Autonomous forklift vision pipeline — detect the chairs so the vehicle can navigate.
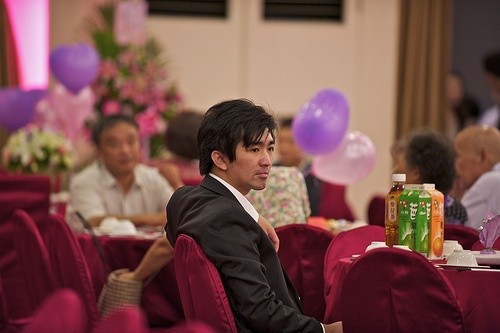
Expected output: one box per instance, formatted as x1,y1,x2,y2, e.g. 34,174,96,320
0,164,500,333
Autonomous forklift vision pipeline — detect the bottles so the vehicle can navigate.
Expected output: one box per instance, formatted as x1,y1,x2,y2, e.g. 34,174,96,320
399,183,444,260
385,174,406,247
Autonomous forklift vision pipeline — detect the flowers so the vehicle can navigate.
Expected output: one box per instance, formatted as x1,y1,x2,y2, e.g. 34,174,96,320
2,4,189,191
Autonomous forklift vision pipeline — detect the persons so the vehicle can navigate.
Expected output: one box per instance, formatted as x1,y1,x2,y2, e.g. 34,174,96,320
64,114,175,234
391,51,500,232
273,117,320,217
164,100,343,333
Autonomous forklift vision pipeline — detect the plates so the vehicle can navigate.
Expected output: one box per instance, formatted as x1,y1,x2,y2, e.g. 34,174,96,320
438,264,490,269
468,251,500,264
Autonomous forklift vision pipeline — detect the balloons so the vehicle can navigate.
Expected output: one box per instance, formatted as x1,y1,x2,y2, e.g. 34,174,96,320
310,131,376,185
0,43,100,134
292,89,349,156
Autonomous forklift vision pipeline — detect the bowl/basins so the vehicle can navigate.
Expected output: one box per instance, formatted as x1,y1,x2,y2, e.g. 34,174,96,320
446,249,477,266
365,242,388,253
99,217,137,234
443,240,463,256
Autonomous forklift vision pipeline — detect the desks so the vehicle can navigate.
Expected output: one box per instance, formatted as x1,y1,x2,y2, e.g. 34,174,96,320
82,227,182,326
323,257,500,333
